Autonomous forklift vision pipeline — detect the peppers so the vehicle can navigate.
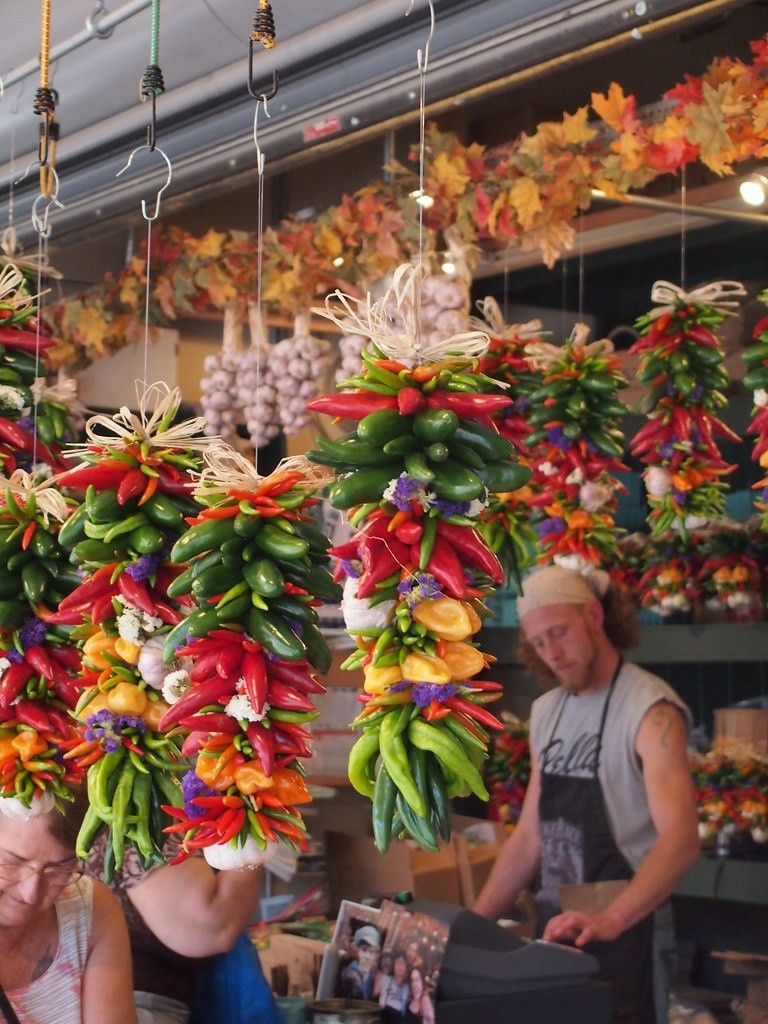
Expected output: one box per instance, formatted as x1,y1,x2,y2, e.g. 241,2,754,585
0,293,768,885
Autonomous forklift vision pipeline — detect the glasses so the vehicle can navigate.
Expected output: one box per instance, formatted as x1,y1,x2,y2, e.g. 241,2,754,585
0,858,85,887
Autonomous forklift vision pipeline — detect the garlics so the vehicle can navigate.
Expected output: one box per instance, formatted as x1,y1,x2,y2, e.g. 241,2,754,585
578,481,608,513
199,278,462,447
644,466,675,496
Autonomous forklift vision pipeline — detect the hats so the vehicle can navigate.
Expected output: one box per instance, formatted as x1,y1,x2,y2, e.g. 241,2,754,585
355,925,379,949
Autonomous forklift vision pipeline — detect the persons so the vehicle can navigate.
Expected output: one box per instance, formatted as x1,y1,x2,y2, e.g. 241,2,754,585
0,782,139,1024
470,554,702,1024
340,925,435,1024
78,822,263,1024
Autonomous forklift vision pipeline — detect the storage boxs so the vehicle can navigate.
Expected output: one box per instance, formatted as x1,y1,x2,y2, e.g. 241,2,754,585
328,808,510,924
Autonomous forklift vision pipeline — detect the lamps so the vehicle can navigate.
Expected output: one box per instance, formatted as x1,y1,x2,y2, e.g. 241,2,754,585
738,165,768,205
